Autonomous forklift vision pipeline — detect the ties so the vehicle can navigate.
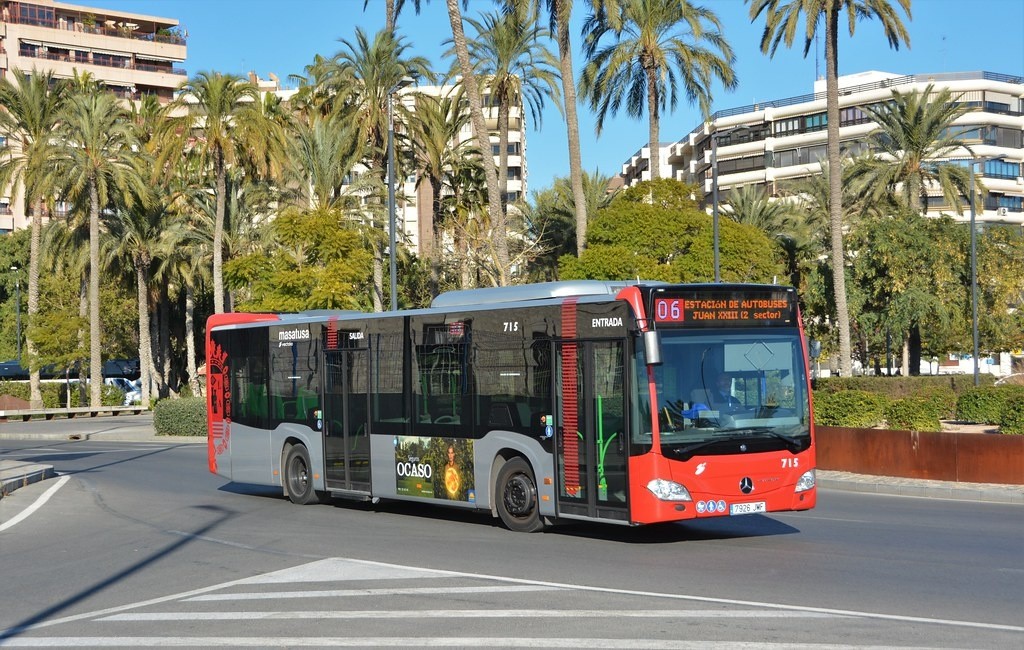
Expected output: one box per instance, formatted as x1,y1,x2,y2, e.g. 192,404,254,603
725,395,730,403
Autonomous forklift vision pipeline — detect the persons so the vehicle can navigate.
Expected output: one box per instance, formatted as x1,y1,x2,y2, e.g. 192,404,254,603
707,370,741,413
444,445,460,497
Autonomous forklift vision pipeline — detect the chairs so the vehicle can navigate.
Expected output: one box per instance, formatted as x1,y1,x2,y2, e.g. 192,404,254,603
405,416,458,424
488,403,513,427
249,382,319,420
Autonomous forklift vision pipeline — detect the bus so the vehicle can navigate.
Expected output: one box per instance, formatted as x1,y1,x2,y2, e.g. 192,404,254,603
207,280,821,535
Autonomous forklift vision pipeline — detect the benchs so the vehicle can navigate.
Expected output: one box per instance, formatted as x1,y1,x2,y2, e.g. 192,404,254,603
0,405,149,422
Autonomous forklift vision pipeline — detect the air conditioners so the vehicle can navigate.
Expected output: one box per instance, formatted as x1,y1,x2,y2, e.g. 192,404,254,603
998,208,1008,215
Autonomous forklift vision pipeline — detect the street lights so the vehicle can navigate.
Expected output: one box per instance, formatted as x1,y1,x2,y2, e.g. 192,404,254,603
970,153,1010,384
710,124,750,283
11,266,21,360
385,76,416,311
